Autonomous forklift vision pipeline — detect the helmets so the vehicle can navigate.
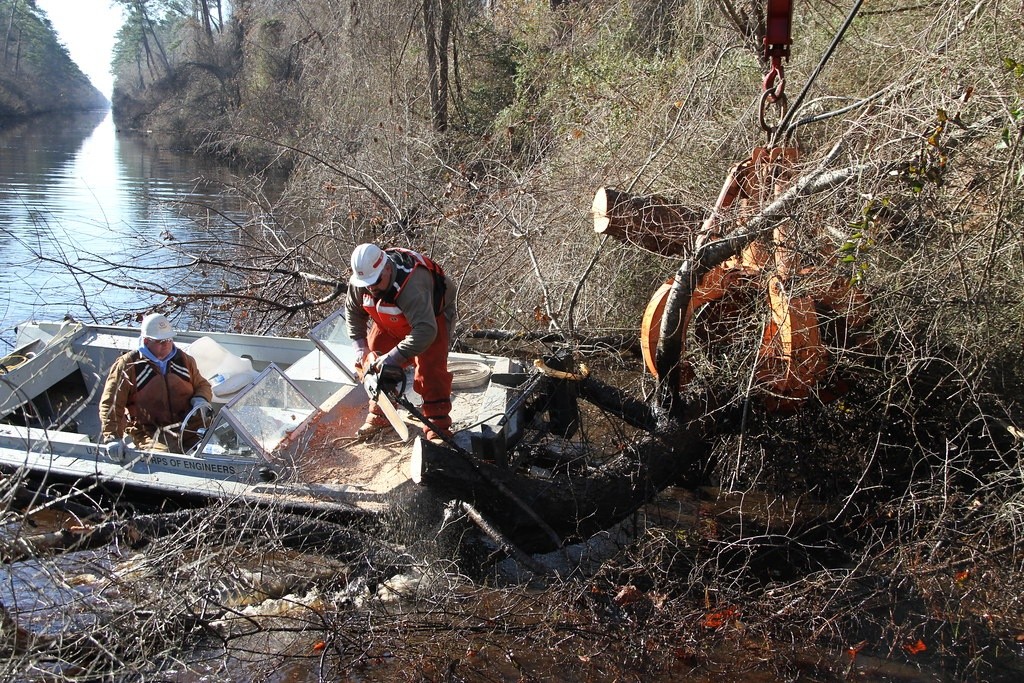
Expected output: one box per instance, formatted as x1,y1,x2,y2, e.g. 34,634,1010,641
349,243,388,288
141,311,177,341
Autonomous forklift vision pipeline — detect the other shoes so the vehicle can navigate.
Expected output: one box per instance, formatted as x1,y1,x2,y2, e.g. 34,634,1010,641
428,429,454,443
359,415,390,438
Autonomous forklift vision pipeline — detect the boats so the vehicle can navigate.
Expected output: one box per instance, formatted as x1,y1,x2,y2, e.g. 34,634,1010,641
0,315,524,524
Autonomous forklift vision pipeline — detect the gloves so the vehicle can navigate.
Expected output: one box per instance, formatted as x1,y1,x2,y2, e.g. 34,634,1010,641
353,338,370,367
106,441,127,462
191,396,212,417
373,346,408,371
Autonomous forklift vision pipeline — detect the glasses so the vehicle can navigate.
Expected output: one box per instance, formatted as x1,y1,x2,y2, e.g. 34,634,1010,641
370,276,383,288
147,338,173,345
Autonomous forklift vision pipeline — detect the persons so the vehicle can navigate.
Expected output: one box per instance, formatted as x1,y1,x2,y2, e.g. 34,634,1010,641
345,243,455,445
99,313,213,462
